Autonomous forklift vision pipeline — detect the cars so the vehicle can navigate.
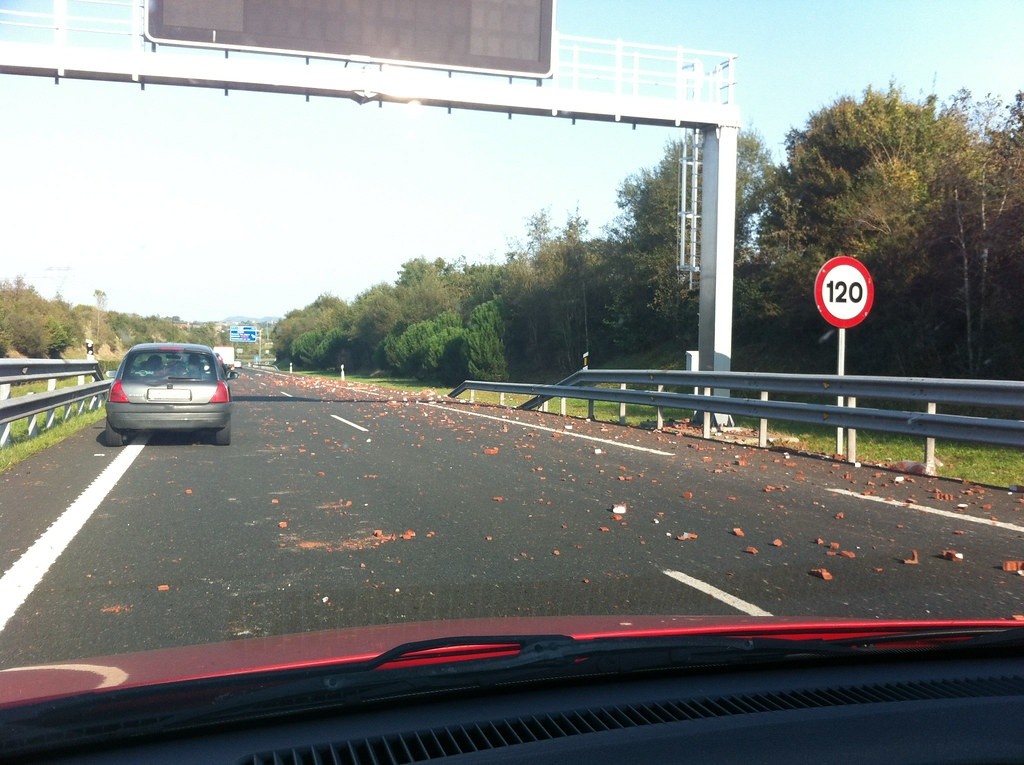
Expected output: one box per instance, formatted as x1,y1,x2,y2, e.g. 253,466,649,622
105,342,239,446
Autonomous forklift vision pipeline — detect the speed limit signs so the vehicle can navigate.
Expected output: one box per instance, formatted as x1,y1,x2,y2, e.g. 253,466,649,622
814,255,875,329
